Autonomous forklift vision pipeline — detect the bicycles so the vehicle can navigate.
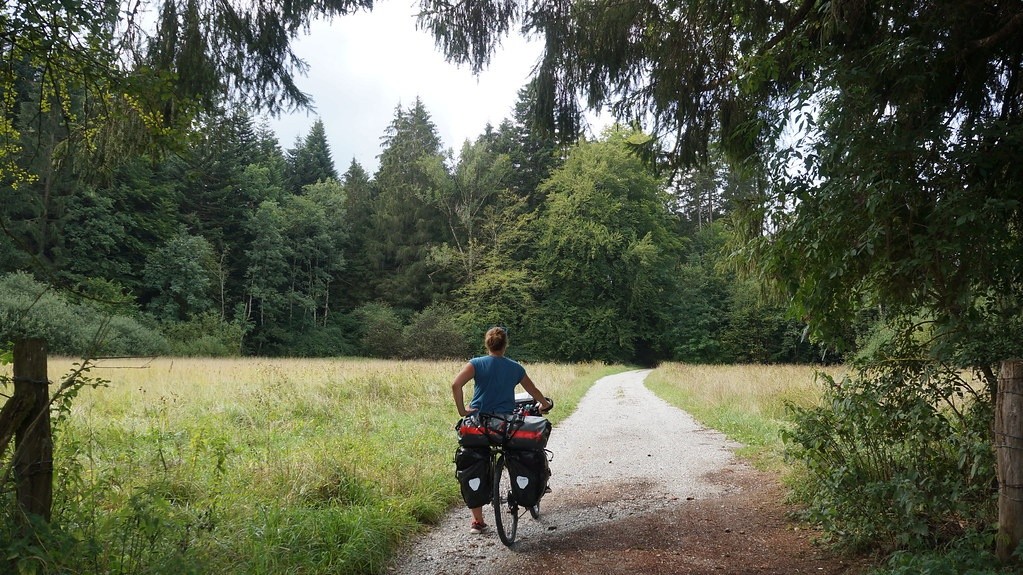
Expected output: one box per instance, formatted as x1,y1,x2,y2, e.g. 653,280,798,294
488,397,555,547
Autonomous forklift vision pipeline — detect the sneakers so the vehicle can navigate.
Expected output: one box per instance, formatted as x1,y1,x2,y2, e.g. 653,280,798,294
545,486,552,493
471,521,489,534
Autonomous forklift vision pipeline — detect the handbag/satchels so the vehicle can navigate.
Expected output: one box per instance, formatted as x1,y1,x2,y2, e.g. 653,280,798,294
452,415,552,511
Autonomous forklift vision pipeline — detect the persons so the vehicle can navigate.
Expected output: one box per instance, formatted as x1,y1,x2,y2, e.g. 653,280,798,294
452,327,552,535
